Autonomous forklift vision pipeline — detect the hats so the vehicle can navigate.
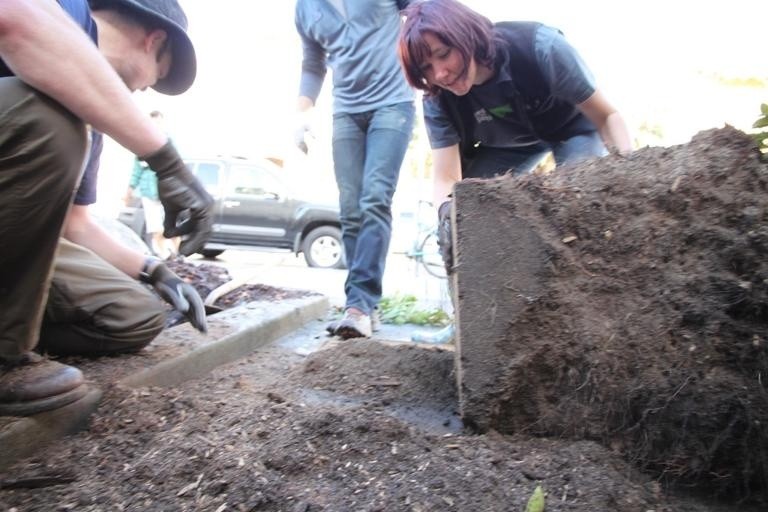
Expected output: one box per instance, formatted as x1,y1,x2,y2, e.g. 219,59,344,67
92,0,196,95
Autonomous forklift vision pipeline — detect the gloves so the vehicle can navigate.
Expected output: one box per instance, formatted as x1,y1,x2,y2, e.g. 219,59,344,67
138,256,208,334
436,200,452,274
138,135,215,257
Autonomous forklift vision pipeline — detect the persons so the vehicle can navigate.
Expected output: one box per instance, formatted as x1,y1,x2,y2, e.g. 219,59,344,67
399,0,634,300
0,0,220,416
122,110,187,262
294,0,416,339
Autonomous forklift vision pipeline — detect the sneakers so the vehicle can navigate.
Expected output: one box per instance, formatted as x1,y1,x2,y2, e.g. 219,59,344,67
0,353,89,416
326,309,380,339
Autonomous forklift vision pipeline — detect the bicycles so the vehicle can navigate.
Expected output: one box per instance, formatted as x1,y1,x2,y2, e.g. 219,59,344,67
404,198,448,281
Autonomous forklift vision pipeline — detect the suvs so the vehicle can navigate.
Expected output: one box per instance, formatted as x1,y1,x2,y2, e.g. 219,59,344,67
137,155,348,270
